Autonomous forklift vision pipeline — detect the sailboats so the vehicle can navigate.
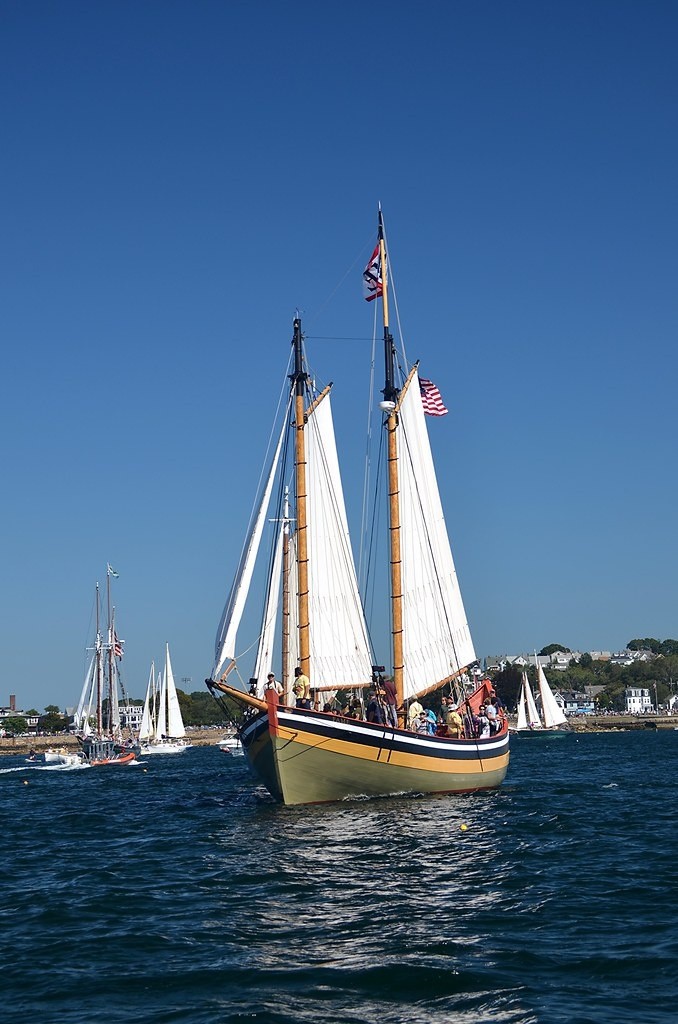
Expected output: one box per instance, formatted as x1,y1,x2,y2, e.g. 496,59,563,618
135,640,195,756
506,648,574,736
73,560,143,759
205,195,508,804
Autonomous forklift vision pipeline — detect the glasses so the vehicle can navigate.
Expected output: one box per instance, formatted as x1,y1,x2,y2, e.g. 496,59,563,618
269,676,274,677
420,714,425,715
480,709,485,711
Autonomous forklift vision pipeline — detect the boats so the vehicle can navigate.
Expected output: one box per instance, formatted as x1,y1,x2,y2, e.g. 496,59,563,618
25,758,42,763
90,751,135,766
214,729,243,754
59,752,88,766
42,746,72,762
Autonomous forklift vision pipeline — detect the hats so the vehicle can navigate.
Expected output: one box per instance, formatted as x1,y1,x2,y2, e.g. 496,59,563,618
367,691,375,696
449,704,459,711
448,696,454,700
295,667,303,674
420,711,426,714
383,674,391,682
268,672,275,676
490,689,496,693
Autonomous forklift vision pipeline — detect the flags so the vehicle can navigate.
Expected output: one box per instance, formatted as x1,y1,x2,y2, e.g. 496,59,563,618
114,644,125,662
419,376,448,417
363,244,389,302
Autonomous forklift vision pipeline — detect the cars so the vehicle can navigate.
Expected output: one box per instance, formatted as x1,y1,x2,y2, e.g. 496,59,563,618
182,723,242,733
0,728,74,739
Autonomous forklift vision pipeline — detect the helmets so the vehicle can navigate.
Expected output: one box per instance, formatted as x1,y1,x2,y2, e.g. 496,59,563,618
410,695,418,702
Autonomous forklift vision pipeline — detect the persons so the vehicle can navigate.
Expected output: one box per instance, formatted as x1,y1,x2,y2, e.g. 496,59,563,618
292,667,311,710
29,747,38,762
263,671,284,705
324,688,504,740
